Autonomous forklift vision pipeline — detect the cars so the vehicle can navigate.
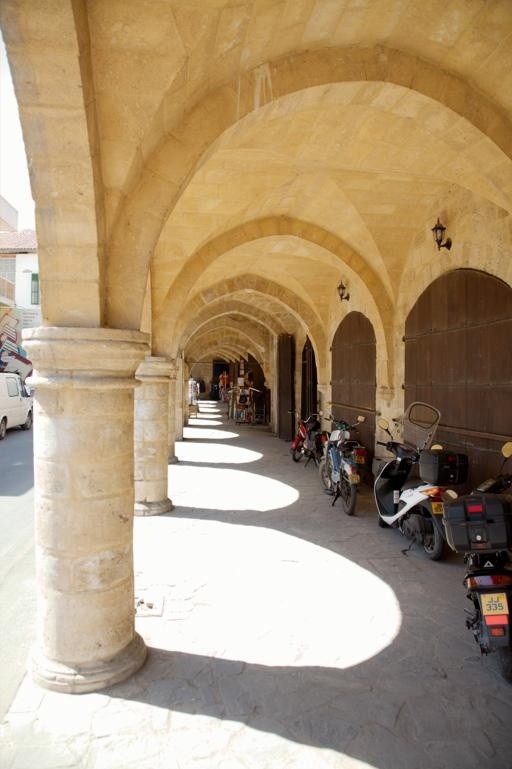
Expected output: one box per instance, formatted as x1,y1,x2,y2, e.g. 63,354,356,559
0,372,32,438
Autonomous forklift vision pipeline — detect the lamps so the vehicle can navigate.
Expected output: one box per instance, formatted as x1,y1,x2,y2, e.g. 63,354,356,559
336,280,349,303
431,217,452,252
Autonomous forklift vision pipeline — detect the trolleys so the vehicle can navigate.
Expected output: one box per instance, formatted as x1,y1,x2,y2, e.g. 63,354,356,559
228,388,259,425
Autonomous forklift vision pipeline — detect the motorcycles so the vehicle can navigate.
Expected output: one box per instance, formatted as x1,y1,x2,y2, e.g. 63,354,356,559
318,410,366,514
288,409,330,466
374,419,469,560
441,441,511,683
